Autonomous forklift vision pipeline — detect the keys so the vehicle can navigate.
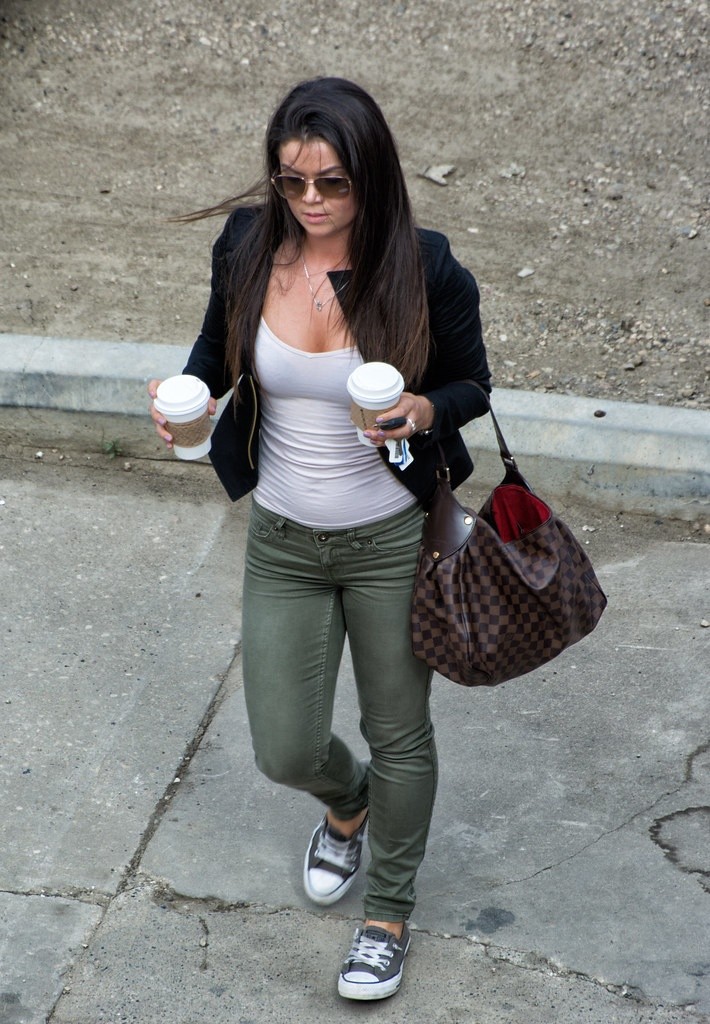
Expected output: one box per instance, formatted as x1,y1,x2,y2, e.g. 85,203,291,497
372,417,406,430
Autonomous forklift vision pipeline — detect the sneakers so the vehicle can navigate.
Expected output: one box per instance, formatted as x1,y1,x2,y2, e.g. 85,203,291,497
338,921,411,1000
303,759,373,907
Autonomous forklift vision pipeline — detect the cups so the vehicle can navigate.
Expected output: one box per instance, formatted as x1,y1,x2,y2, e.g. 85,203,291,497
347,362,405,448
154,376,211,460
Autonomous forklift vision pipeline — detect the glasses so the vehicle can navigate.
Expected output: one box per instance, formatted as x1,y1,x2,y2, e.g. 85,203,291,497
271,166,353,200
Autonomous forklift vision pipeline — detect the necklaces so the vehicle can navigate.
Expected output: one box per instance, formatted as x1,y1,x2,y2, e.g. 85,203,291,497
301,254,350,311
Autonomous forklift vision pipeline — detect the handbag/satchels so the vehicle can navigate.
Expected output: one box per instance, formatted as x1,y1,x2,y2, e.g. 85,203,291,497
410,379,607,688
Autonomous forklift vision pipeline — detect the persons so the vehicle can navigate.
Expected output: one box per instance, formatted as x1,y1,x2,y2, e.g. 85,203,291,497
148,78,493,1001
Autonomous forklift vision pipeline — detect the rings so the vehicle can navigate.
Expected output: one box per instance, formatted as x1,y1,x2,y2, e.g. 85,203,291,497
408,419,416,431
